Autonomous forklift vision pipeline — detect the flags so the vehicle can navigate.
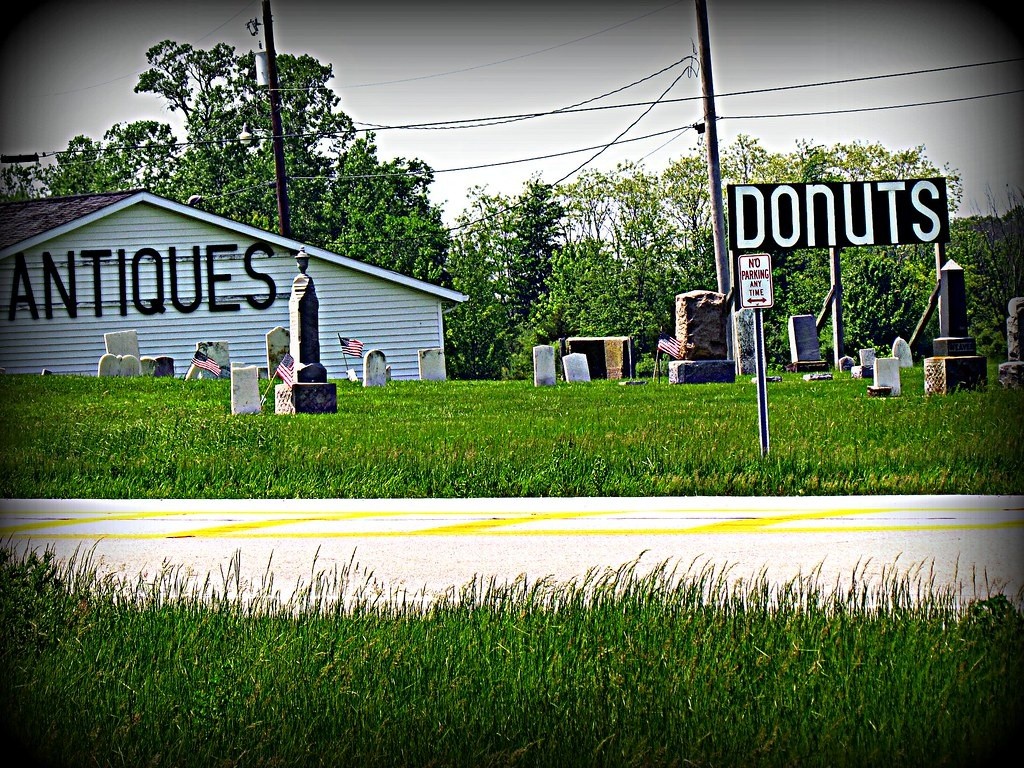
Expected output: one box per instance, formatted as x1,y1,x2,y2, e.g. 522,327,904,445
191,351,222,377
658,332,681,359
276,353,294,388
340,337,364,358
